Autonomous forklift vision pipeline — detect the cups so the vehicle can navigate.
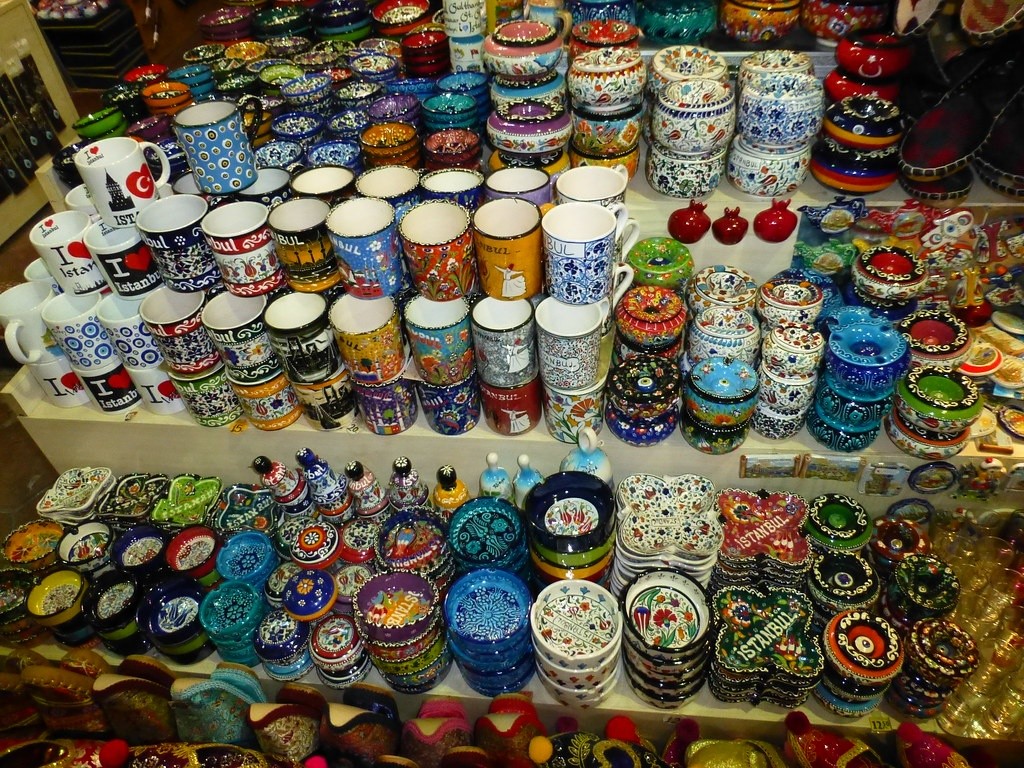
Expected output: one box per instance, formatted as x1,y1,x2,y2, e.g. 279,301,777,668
0,101,641,446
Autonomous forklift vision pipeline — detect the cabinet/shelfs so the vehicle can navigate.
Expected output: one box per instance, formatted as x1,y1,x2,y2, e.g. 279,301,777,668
0,134,1024,768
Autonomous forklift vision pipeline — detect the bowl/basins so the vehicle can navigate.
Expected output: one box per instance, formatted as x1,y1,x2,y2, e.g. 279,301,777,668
49,0,910,200
0,426,980,721
